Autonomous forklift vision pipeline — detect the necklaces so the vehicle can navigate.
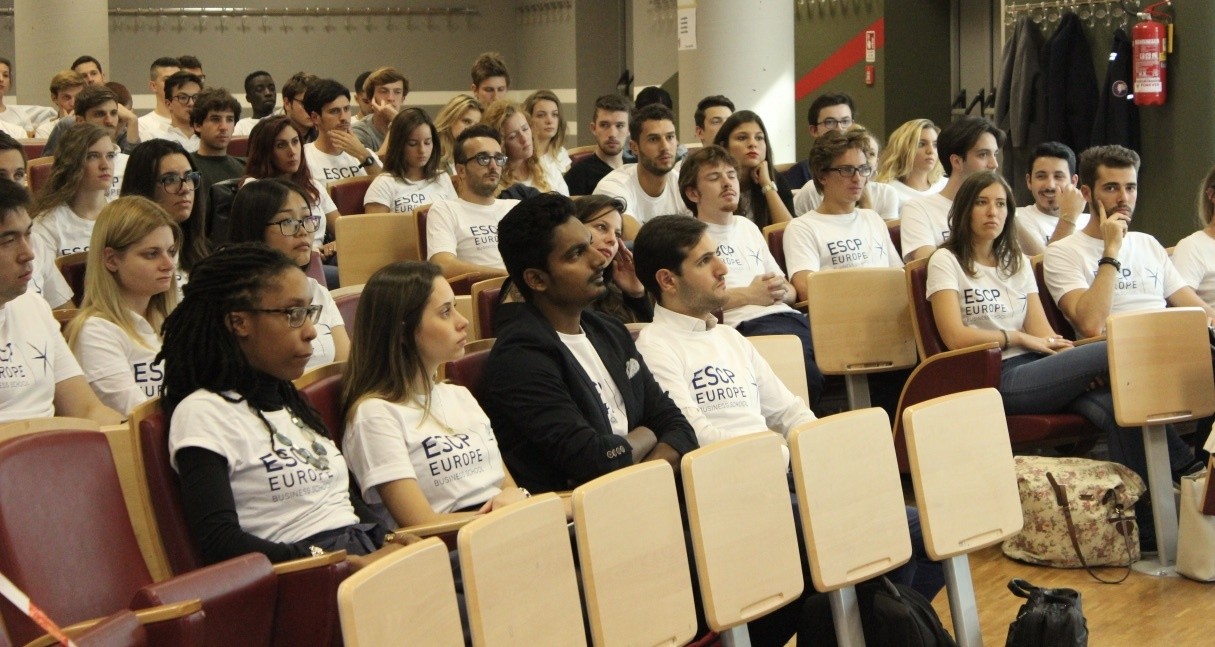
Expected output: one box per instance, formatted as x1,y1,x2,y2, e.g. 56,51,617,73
419,381,454,433
250,407,330,470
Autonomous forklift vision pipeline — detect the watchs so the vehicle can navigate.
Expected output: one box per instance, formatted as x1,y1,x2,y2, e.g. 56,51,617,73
519,487,531,498
359,156,374,168
761,181,779,193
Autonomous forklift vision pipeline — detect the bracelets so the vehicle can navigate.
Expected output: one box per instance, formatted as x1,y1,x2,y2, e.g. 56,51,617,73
999,329,1009,351
385,533,395,541
1097,257,1121,271
309,545,324,557
1060,213,1075,226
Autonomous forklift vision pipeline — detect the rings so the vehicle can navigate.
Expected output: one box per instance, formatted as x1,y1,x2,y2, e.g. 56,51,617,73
1048,338,1054,342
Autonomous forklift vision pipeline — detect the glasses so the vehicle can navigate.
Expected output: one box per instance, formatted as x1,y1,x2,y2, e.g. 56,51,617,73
152,171,201,194
822,166,872,177
171,93,199,104
229,304,323,328
465,154,508,166
816,119,854,129
265,216,321,236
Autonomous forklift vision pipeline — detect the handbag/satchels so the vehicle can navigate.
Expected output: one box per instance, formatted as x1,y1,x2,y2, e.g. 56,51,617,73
1006,579,1089,647
1176,471,1215,582
1002,456,1148,583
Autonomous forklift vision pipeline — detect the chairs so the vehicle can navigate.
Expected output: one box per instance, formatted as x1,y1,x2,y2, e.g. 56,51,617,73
1,108,1215,647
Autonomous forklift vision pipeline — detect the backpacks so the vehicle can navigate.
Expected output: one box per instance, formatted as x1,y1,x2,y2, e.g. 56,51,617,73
798,575,955,647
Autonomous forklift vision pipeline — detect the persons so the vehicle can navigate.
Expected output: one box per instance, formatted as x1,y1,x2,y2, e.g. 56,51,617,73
0,50,1215,647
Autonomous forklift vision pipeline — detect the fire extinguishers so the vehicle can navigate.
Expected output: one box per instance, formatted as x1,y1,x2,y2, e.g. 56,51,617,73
1120,0,1168,106
